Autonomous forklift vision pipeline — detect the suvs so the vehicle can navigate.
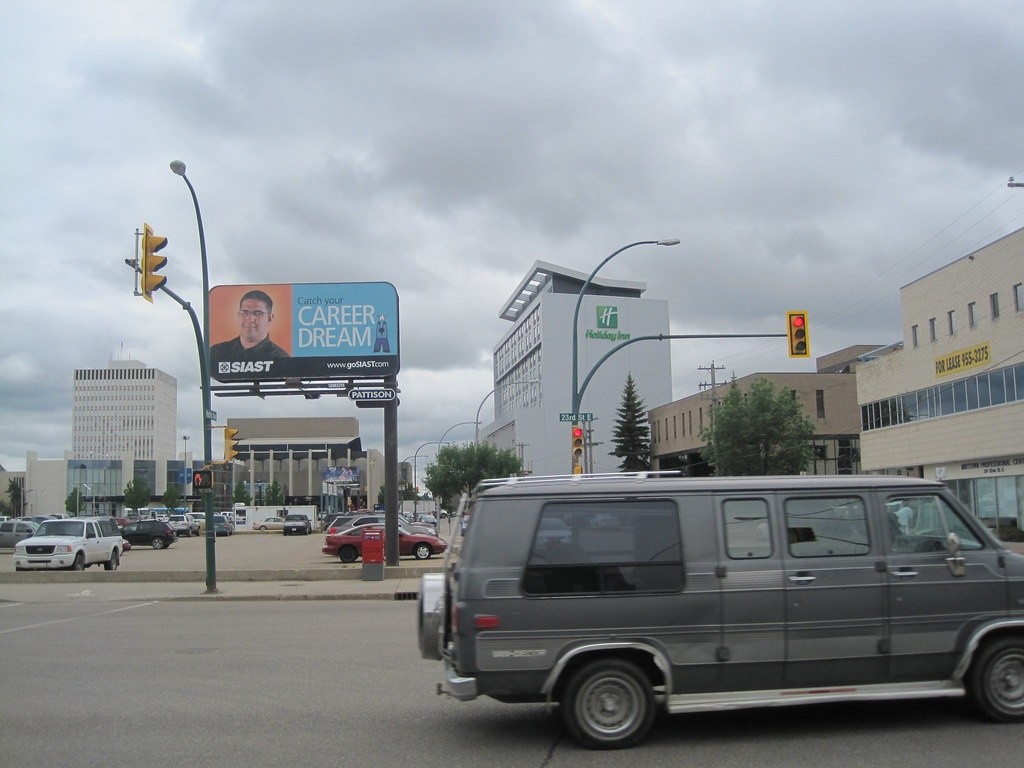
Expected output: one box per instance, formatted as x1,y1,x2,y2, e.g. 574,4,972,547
417,467,1024,751
117,519,179,549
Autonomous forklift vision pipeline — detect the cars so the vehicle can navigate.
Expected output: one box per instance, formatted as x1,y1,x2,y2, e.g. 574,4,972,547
318,511,448,563
0,513,131,548
155,511,235,538
253,514,313,536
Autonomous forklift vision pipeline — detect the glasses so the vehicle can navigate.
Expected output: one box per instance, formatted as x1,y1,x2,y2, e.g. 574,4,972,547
238,309,269,317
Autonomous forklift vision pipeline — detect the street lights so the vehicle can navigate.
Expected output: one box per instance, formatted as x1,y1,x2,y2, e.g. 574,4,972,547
436,421,483,534
181,434,190,512
569,239,683,423
474,379,540,447
401,454,429,513
169,160,210,403
414,442,450,521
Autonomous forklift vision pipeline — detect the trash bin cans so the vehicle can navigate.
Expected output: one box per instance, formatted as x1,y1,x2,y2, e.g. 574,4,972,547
361,530,385,581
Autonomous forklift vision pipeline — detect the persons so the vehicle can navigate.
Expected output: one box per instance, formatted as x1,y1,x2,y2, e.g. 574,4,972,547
897,504,914,535
759,521,769,536
300,516,304,520
211,289,289,360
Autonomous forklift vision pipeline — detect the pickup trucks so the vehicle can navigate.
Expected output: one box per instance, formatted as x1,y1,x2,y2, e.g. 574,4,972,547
13,520,123,572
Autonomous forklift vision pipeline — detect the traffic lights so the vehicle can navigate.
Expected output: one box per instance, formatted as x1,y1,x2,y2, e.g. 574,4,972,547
572,425,585,458
193,471,212,489
142,223,168,303
224,428,240,462
788,309,810,357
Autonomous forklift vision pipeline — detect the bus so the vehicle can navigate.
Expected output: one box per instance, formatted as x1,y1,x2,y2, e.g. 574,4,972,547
125,507,189,519
